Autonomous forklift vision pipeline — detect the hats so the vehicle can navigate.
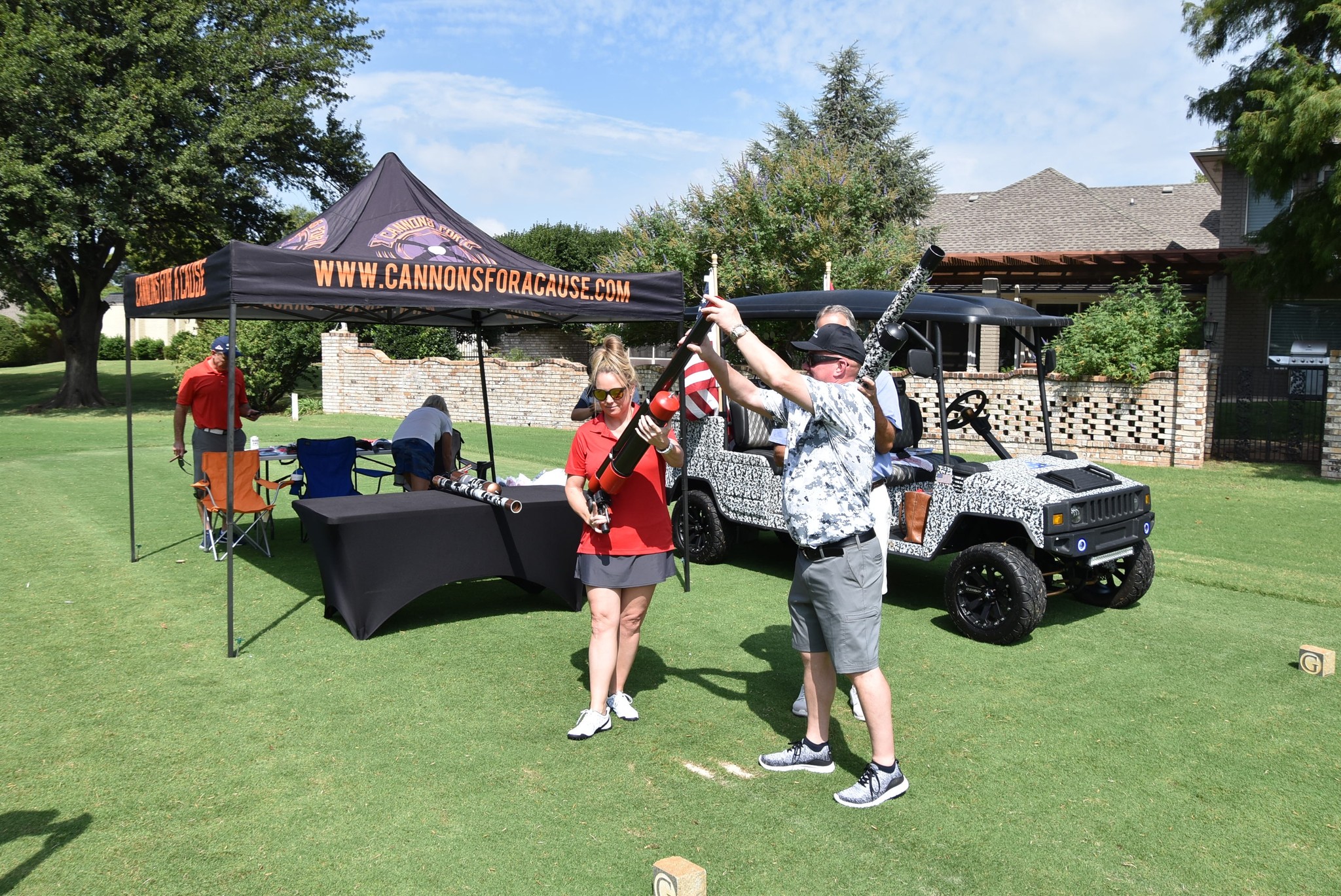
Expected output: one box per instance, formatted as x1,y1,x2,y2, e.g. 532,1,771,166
790,323,866,366
211,336,246,359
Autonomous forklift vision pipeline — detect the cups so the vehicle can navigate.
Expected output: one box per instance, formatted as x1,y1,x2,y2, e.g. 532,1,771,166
291,474,303,481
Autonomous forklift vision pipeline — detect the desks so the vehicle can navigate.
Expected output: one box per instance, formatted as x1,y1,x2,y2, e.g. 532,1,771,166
291,485,589,640
244,448,392,539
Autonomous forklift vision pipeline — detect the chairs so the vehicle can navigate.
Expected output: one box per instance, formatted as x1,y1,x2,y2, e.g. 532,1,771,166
190,449,295,561
289,436,396,542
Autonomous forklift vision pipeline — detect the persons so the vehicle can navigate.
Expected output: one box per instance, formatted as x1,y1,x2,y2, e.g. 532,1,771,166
676,294,907,808
766,305,903,722
391,395,453,492
564,334,685,740
173,336,261,549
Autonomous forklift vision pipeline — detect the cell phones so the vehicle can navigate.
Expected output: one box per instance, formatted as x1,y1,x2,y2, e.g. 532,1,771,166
246,412,265,417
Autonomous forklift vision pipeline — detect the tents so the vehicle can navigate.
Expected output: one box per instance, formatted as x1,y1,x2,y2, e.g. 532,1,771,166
119,152,703,661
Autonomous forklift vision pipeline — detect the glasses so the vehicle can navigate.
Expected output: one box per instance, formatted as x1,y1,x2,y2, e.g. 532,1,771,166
178,456,193,475
592,383,629,401
799,352,849,367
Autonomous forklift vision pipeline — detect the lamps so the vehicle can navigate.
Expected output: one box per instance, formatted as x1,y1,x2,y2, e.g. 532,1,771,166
1202,312,1219,349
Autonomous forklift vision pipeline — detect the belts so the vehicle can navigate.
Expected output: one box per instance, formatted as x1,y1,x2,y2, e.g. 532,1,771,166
194,422,240,435
801,527,877,560
871,478,886,492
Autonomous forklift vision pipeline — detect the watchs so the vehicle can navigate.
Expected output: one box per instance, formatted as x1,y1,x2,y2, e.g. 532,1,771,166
730,323,751,344
656,438,673,455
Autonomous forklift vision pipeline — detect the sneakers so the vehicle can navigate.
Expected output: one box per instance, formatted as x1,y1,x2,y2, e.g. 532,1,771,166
758,739,836,773
567,706,612,741
607,690,639,721
792,683,808,716
833,760,910,809
216,527,243,546
199,529,216,549
849,684,867,722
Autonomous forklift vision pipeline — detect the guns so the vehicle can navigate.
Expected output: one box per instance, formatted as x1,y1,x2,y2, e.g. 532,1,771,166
586,295,727,534
430,472,523,514
858,244,945,391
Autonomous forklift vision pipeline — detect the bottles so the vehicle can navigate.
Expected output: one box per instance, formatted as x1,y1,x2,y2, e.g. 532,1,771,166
250,436,259,450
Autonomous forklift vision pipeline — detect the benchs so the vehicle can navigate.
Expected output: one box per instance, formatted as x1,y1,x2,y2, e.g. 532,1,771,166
882,377,967,485
729,399,785,476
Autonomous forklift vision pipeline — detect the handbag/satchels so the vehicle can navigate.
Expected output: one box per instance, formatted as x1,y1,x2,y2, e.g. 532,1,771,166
903,490,933,544
